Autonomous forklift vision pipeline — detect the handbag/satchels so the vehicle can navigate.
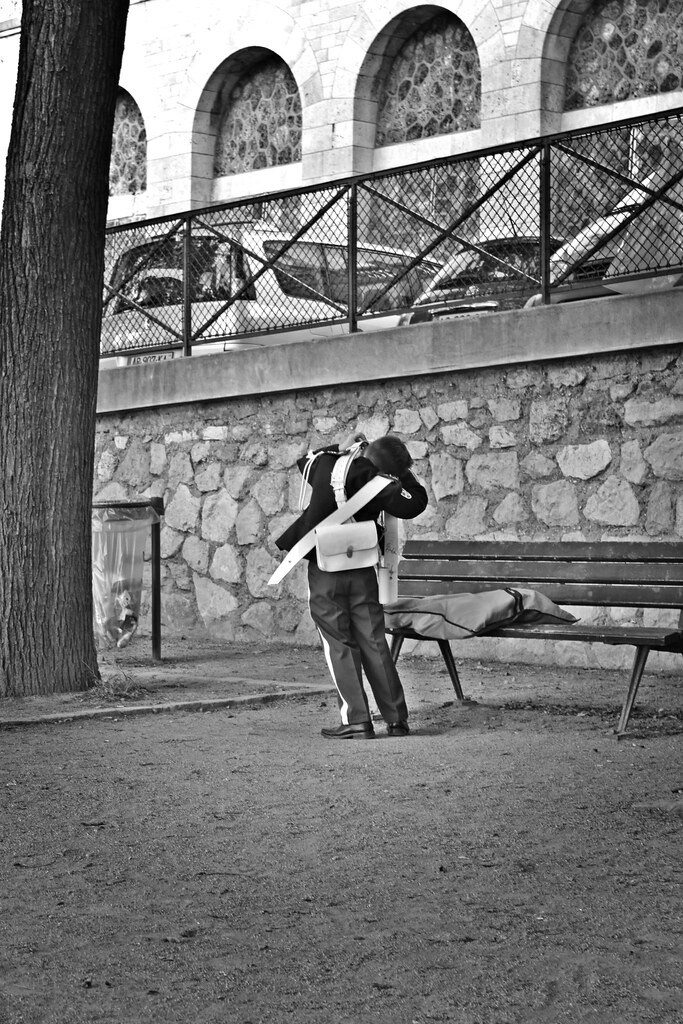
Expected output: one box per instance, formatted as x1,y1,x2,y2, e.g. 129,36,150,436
315,517,382,572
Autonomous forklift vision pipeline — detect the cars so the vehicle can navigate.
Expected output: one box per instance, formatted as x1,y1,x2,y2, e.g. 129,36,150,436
521,170,683,309
397,235,570,328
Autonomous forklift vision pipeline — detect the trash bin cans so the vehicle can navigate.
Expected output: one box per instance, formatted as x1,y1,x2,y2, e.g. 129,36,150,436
92,498,152,649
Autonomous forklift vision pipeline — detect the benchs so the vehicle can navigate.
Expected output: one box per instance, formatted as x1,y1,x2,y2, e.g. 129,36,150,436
386,538,683,737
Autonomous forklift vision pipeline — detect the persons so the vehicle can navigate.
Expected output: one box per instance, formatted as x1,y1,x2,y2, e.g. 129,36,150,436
274,433,429,739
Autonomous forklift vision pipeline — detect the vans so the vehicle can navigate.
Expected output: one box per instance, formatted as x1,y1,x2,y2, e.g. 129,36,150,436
99,219,445,366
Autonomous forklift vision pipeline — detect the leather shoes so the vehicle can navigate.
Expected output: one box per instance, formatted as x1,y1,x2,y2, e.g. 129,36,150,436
387,719,409,735
321,721,375,740
